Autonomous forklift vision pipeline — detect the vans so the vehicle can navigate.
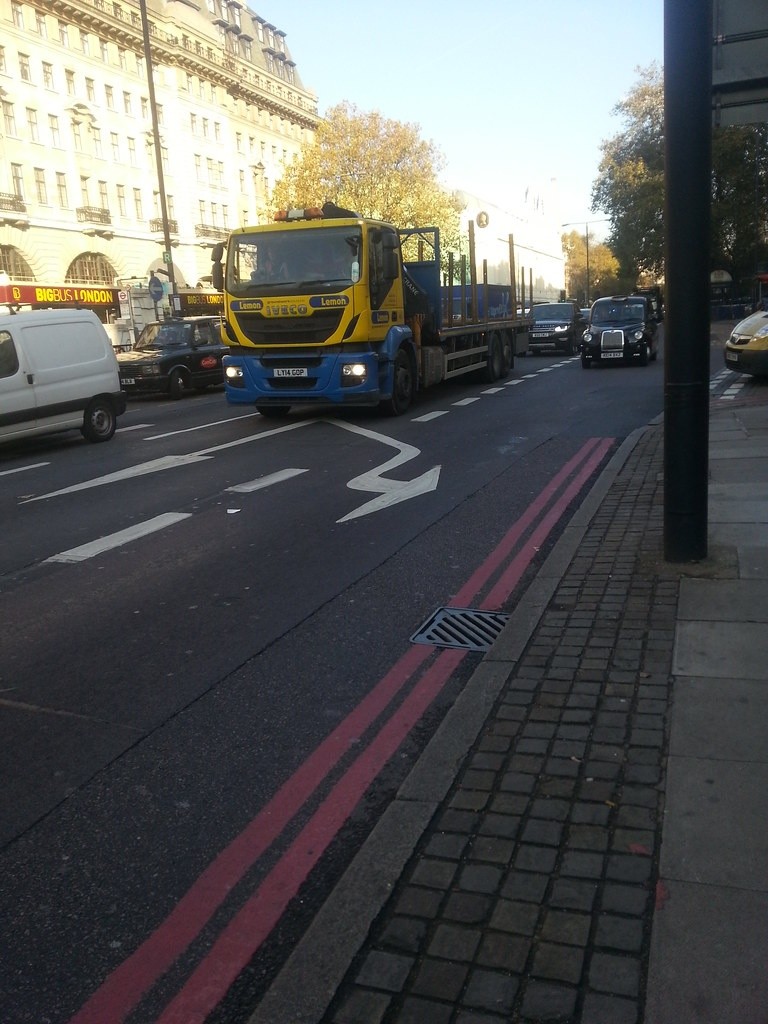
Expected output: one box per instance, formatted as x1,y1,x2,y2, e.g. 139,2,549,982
0,308,129,446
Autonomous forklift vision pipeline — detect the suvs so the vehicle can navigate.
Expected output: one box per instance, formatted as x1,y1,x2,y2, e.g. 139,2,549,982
116,315,230,401
578,294,660,370
524,302,587,357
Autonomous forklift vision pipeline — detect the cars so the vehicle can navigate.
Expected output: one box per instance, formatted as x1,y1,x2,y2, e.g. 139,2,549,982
723,303,768,377
516,298,593,326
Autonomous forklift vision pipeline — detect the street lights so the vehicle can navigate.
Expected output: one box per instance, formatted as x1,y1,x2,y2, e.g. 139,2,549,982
561,218,611,308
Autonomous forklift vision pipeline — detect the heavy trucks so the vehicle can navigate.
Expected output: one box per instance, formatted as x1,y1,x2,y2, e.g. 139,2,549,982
210,201,535,419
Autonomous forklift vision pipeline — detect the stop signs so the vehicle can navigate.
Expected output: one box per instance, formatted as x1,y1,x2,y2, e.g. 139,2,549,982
118,290,128,301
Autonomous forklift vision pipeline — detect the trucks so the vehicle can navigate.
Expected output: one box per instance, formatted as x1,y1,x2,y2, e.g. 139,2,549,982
634,285,663,323
